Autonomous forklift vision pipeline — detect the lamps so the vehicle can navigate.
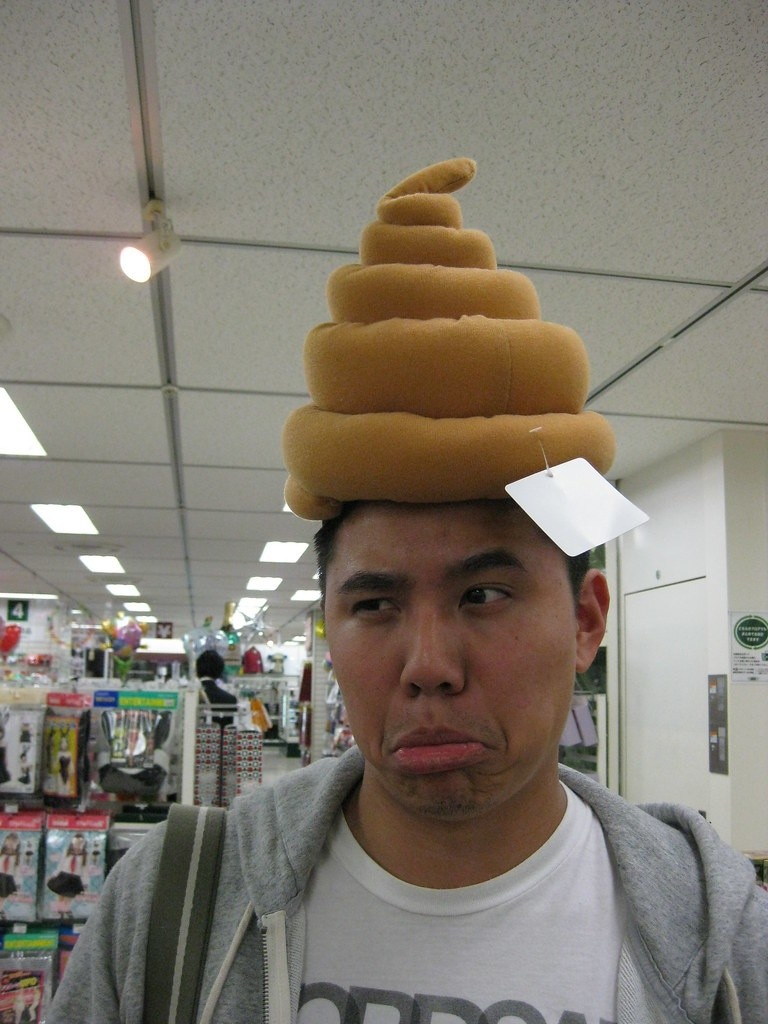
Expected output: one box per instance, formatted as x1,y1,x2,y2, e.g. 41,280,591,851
119,200,183,284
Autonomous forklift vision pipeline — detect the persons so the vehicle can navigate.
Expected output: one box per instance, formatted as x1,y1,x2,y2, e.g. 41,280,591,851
44,499,768,1024
